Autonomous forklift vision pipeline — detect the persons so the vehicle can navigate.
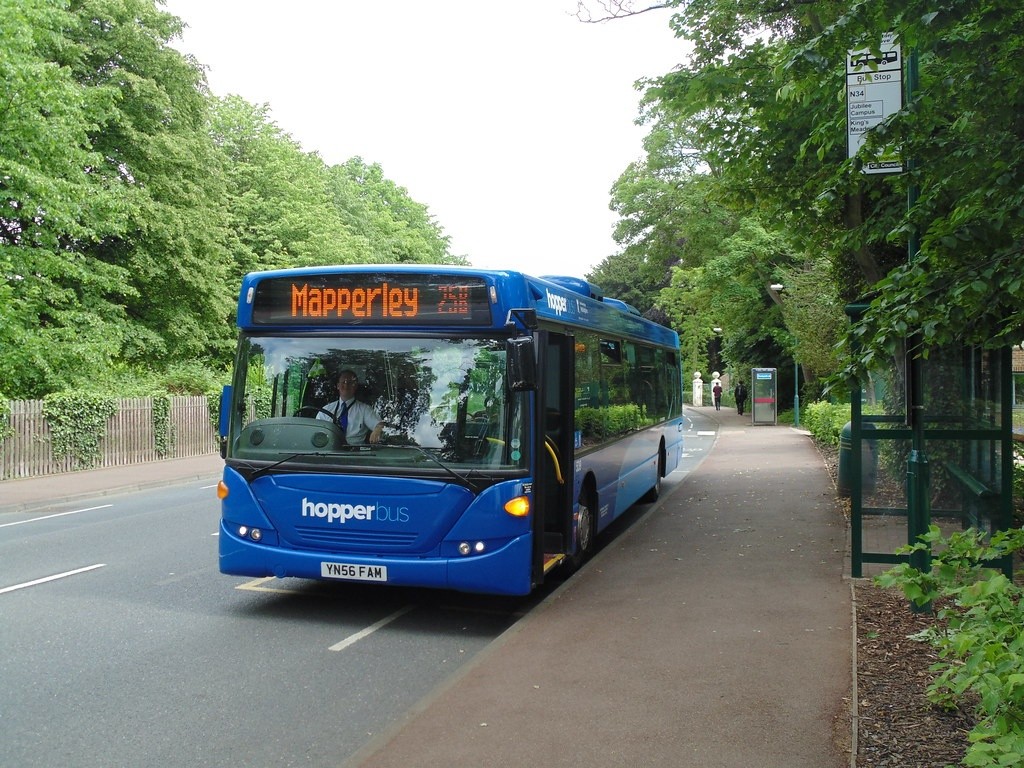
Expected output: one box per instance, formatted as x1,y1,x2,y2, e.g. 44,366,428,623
315,370,384,445
713,382,722,410
735,381,748,416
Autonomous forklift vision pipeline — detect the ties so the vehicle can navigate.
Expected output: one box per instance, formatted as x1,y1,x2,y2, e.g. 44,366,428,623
339,402,348,436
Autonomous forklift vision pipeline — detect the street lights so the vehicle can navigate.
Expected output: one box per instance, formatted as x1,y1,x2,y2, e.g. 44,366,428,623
770,284,800,427
713,327,732,398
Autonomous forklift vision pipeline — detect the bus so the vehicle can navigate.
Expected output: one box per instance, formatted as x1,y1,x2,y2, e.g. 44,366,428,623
218,265,685,596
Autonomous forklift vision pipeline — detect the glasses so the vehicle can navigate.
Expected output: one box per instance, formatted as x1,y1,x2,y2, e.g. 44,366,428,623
339,380,357,388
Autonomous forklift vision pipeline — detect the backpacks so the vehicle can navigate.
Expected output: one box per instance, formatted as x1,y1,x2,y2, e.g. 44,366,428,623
740,386,748,399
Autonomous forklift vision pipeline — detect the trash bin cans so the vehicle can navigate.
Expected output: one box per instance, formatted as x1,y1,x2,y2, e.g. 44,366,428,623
836,419,879,502
